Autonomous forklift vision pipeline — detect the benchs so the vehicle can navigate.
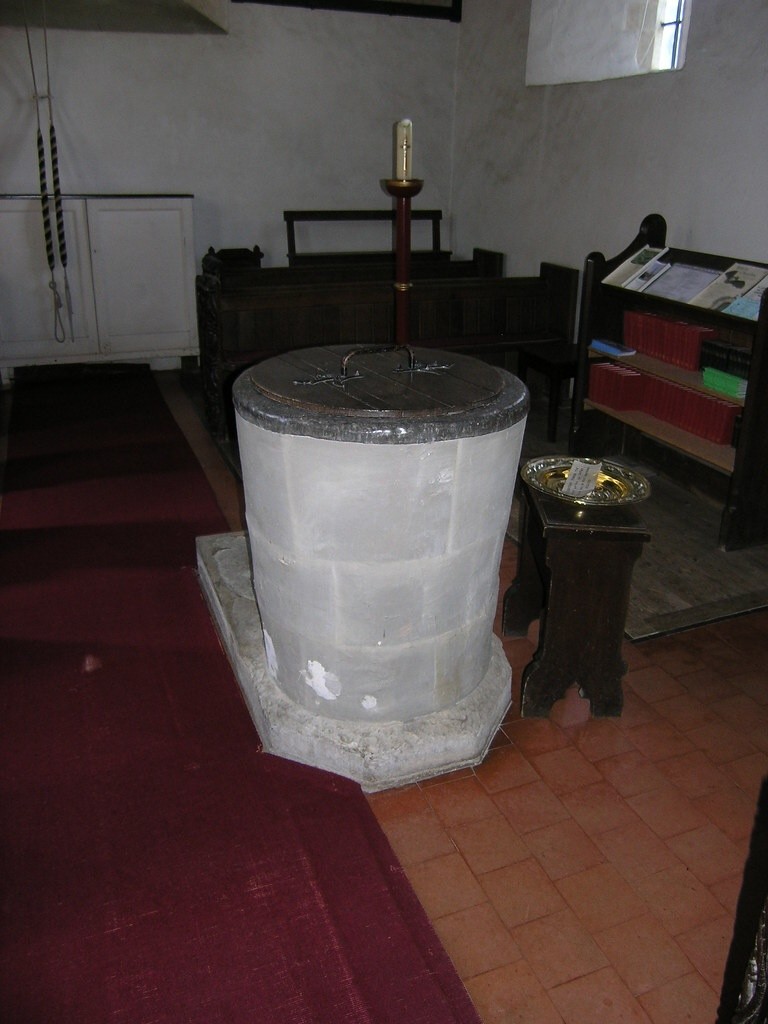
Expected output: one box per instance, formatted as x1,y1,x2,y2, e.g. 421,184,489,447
195,248,579,447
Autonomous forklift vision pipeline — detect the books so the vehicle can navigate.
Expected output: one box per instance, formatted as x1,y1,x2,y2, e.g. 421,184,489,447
590,243,768,444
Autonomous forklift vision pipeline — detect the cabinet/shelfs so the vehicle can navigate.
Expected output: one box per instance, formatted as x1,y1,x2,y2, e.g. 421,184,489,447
569,212,768,551
0,194,201,388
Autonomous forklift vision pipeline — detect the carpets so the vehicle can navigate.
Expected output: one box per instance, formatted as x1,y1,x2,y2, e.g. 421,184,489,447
1,364,768,818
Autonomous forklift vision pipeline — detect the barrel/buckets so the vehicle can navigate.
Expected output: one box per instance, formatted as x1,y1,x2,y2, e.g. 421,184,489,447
234,342,528,721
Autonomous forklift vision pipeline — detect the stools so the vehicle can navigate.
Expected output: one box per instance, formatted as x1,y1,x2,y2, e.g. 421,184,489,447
501,457,648,719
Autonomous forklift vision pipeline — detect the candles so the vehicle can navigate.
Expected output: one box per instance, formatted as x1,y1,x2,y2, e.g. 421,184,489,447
397,118,413,180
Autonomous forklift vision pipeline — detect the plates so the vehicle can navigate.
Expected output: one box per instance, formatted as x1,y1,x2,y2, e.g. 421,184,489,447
521,455,651,506
